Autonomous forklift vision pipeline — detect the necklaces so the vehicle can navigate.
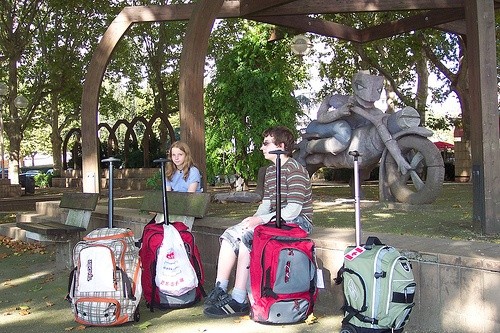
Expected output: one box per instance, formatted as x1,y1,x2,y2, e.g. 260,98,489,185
177,168,184,173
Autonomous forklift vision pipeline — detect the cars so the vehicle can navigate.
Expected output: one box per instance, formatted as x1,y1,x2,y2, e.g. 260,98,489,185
0,169,8,179
19,169,53,182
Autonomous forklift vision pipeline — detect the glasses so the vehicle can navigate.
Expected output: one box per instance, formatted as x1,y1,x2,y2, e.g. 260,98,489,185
261,141,273,147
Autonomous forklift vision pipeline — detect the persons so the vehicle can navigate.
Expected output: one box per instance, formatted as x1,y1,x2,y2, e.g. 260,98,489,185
135,141,202,248
202,127,313,319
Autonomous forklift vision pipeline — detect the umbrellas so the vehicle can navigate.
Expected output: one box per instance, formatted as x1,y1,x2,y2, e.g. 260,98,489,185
434,141,454,150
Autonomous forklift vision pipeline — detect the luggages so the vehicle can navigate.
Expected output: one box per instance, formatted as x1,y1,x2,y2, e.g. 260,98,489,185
334,151,416,333
135,159,207,311
64,158,143,326
247,151,319,325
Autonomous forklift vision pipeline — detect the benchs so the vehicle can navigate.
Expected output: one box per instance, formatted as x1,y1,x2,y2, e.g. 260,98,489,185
16,191,99,271
141,190,211,232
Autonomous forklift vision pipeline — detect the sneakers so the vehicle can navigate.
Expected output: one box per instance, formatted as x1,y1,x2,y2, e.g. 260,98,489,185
202,282,229,308
204,294,251,319
